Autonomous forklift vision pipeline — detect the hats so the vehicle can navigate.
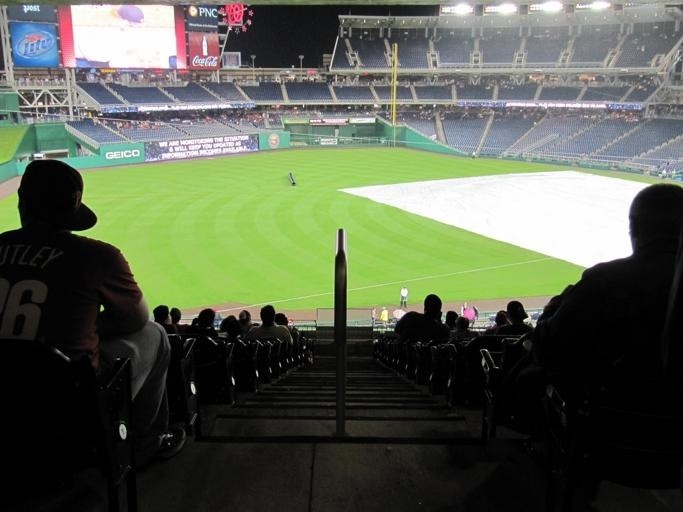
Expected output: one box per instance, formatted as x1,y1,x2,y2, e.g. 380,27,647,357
17,159,97,231
507,301,528,319
238,310,250,325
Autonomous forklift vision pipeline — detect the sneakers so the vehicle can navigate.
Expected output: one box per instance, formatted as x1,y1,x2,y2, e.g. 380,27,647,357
134,425,187,472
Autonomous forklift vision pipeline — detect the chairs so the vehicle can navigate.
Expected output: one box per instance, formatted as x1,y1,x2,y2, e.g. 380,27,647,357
0,331,314,512
373,337,683,512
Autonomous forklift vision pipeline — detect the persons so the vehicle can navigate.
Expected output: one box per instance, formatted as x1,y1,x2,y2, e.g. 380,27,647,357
0,68,683,131
374,283,550,407
529,182,682,511
151,302,301,389
1,159,190,511
